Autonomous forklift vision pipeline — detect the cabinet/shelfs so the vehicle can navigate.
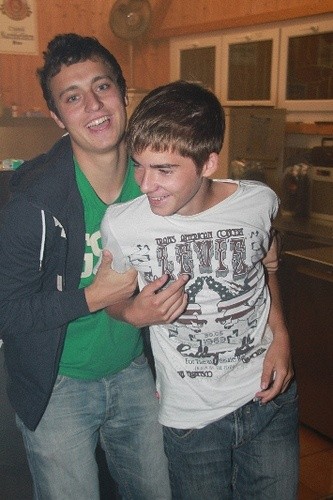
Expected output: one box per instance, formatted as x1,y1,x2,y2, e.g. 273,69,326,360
168,11,333,125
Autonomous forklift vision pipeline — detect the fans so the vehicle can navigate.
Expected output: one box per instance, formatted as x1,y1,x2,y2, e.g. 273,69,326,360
108,0,155,93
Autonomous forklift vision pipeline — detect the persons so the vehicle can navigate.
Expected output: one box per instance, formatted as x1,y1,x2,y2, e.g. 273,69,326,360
0,32,282,499
100,81,300,499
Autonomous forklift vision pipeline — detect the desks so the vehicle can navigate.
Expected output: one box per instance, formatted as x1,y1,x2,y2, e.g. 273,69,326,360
268,216,331,442
